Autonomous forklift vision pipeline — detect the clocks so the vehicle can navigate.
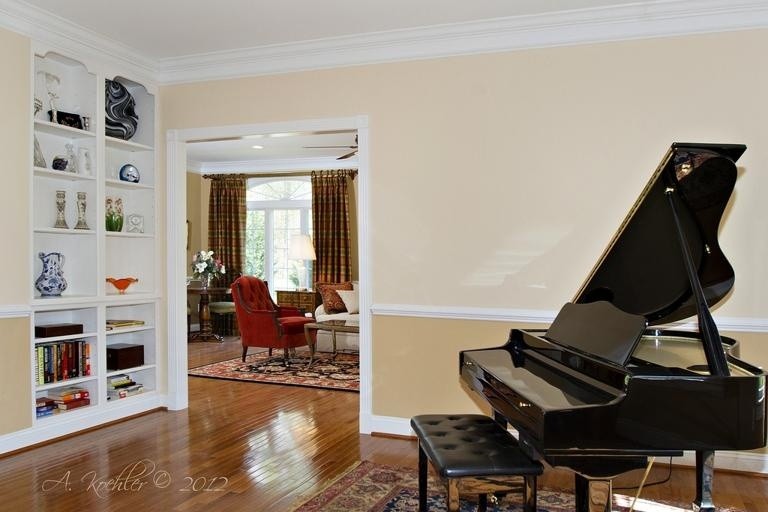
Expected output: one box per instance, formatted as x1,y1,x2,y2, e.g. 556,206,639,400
127,214,144,233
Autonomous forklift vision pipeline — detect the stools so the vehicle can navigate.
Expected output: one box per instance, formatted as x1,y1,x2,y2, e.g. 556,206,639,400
208,301,236,342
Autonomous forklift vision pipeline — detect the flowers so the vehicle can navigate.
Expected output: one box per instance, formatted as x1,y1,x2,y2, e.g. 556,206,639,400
187,249,226,286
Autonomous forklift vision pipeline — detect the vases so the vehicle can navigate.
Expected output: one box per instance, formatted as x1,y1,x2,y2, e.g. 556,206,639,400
200,275,208,290
75,192,90,230
54,191,69,229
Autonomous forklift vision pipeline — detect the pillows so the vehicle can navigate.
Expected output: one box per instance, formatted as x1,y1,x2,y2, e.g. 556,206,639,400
337,290,360,314
315,282,353,314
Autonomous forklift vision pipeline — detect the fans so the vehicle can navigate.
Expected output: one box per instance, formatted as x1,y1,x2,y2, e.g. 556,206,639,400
336,134,358,160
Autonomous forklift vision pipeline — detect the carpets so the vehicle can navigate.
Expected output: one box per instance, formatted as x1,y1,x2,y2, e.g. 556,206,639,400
285,460,688,512
188,345,360,393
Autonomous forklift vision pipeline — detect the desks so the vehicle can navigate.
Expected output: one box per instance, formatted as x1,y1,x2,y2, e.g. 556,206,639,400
187,287,232,343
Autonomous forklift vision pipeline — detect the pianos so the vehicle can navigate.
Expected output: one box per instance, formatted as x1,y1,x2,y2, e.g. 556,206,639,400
459,143,767,510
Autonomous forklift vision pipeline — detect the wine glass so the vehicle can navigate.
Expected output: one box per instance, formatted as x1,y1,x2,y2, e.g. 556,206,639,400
106,277,138,295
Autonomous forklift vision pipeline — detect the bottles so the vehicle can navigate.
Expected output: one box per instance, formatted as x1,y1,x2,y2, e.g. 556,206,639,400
65,143,91,175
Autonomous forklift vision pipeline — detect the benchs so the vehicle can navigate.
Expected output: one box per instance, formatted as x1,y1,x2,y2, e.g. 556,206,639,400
411,414,544,512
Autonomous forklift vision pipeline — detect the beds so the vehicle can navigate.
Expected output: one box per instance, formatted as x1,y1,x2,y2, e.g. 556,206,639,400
304,281,360,371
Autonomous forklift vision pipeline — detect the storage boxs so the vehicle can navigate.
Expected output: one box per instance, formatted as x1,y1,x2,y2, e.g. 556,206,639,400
106,343,144,370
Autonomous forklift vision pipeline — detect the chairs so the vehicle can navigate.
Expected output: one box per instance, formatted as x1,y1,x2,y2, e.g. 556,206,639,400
231,275,317,362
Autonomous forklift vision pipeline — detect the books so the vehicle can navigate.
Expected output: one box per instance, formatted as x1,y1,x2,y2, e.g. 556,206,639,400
107,373,146,401
33,339,92,419
105,318,146,332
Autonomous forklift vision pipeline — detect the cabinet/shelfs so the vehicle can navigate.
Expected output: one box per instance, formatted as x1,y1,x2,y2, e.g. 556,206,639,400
275,290,316,358
102,32,189,425
29,34,105,449
